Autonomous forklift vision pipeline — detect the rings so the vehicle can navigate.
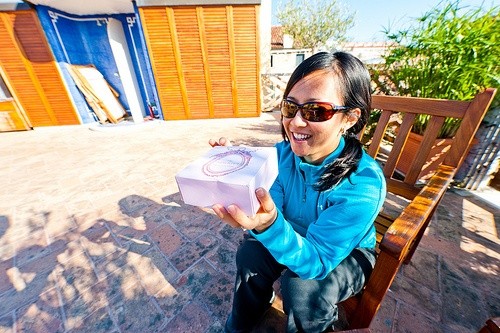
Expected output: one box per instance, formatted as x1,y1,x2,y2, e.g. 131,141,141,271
241,227,247,231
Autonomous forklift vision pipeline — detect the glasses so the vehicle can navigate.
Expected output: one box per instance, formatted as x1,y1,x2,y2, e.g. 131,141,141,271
280,99,352,122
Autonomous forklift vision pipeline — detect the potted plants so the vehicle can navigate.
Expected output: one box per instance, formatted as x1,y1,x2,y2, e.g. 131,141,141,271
369,0,500,181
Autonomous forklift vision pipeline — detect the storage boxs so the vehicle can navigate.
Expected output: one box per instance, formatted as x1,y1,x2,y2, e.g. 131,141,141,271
175,146,279,218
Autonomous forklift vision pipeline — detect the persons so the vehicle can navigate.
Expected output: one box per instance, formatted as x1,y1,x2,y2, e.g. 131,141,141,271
195,50,387,333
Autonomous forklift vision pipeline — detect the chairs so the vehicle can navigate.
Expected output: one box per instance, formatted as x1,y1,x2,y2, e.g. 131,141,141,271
274,87,498,329
